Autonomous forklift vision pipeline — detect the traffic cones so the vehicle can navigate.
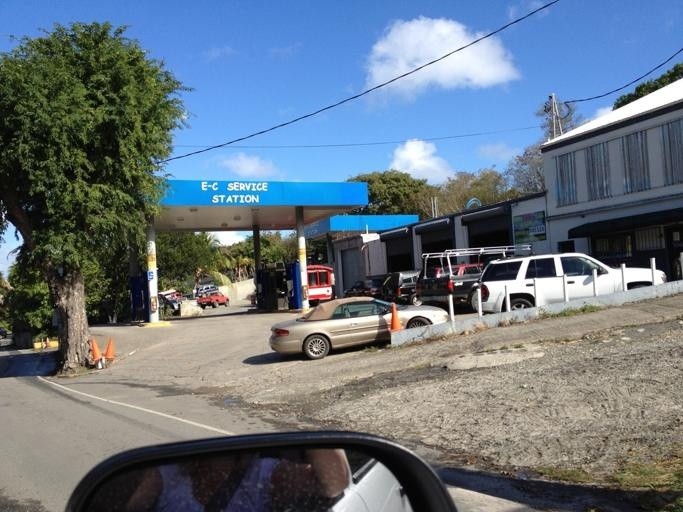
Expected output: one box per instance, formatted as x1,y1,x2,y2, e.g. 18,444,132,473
104,337,115,360
387,302,403,333
44,338,51,349
91,337,102,361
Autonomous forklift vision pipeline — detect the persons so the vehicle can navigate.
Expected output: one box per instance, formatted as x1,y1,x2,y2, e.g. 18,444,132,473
122,444,352,510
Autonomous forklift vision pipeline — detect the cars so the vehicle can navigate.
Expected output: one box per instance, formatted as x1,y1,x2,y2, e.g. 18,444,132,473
198,291,229,308
158,289,184,315
269,297,450,359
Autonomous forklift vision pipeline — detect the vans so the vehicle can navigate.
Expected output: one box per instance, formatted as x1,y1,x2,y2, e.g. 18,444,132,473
198,285,216,296
471,251,667,315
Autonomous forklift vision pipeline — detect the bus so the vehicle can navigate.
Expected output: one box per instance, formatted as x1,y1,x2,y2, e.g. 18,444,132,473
253,266,332,308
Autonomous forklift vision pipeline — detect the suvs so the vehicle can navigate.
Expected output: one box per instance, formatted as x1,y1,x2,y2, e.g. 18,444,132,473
340,264,482,310
